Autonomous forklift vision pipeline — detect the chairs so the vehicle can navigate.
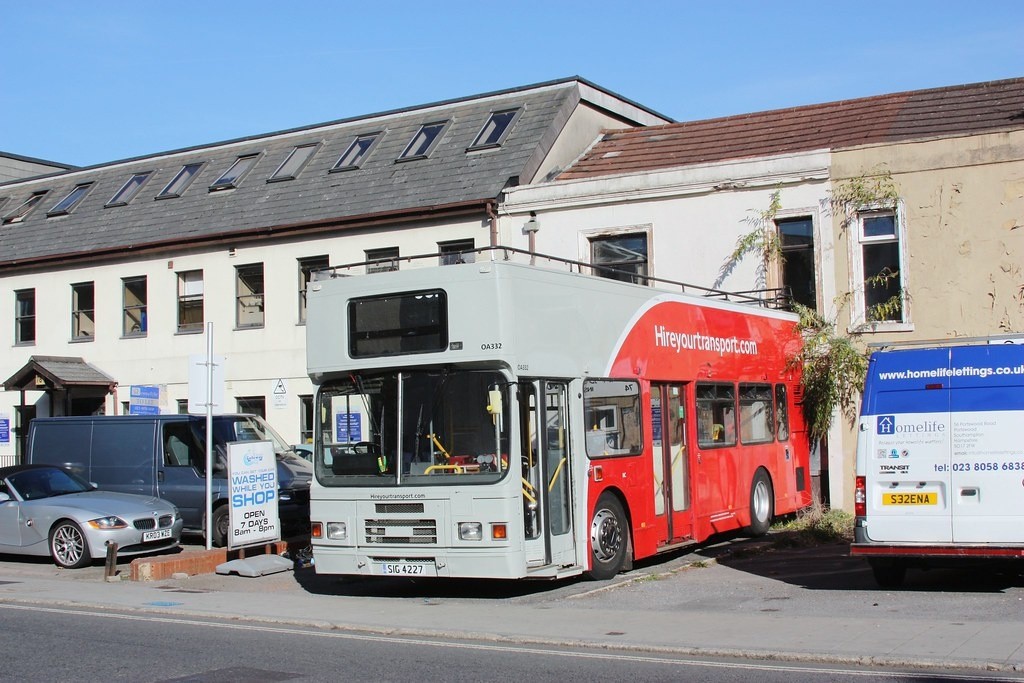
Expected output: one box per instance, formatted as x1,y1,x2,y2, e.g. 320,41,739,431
165,427,201,466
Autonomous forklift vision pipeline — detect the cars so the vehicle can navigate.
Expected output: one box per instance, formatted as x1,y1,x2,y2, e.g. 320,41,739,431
293,443,363,464
0,464,183,569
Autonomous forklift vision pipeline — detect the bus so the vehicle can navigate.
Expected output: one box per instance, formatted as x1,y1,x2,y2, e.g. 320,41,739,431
300,247,814,583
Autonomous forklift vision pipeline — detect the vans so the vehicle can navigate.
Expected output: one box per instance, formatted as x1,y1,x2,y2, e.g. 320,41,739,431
848,332,1024,558
24,411,313,547
